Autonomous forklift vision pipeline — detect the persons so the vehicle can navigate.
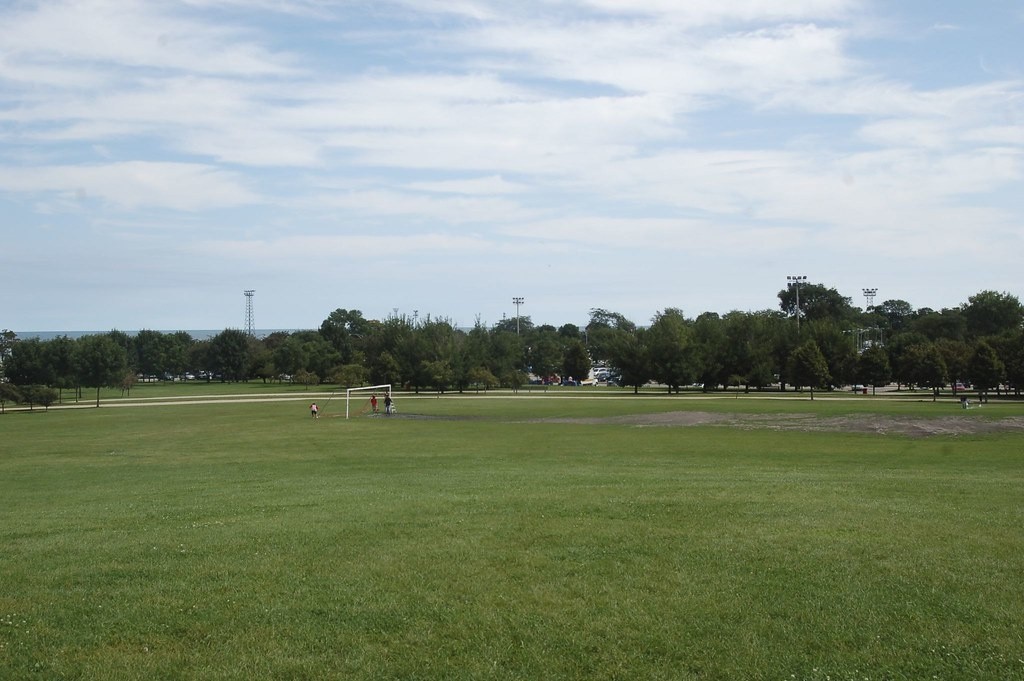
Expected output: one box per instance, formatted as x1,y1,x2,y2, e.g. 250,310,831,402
309,402,319,419
370,394,378,416
385,394,393,414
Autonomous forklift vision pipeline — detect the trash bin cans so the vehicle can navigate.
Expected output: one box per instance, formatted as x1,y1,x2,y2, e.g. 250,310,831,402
863,388,868,394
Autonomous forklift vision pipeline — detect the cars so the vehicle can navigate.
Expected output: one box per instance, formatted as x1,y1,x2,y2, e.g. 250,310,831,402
139,370,222,380
852,385,864,391
952,383,966,391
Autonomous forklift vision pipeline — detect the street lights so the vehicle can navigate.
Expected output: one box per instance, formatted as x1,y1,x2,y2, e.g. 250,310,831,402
785,275,808,337
513,297,525,338
862,288,878,308
